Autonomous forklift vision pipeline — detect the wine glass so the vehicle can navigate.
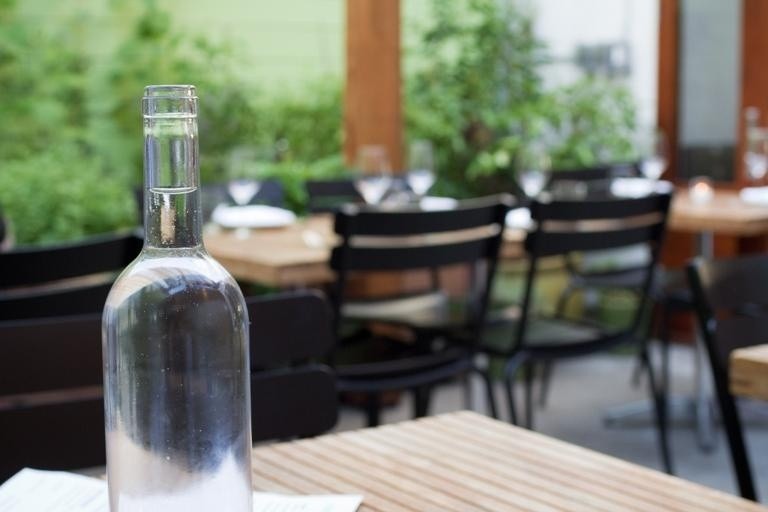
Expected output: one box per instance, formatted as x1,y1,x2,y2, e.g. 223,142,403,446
510,142,553,202
632,128,675,182
225,131,268,215
352,141,437,209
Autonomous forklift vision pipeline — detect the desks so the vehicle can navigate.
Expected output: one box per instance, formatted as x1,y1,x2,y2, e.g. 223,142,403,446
0,408,768,512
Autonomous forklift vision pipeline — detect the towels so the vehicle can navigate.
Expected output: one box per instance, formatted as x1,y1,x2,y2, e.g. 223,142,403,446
0,467,366,512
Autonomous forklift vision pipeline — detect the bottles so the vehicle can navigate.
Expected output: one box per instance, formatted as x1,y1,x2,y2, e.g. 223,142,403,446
97,83,254,512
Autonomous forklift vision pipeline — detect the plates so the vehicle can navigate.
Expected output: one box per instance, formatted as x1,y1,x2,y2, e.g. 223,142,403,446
738,186,768,208
609,178,674,199
209,202,297,230
